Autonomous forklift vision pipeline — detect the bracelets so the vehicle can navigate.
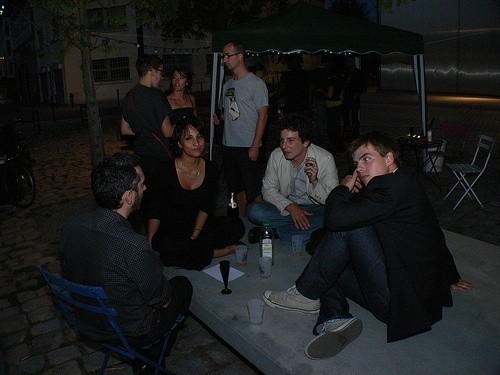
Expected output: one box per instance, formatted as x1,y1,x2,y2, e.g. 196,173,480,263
193,227,203,230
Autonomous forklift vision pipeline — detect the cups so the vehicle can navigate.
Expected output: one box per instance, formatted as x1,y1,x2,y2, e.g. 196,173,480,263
234,245,248,266
258,256,273,279
246,299,264,325
291,236,304,256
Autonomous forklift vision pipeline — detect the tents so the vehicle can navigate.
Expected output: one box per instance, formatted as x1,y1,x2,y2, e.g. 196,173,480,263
209,1,428,161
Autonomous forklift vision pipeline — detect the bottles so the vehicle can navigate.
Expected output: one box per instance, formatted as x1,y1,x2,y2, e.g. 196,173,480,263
260,222,275,267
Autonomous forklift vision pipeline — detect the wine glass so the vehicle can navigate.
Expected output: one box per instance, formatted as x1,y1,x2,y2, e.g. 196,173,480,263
219,261,232,294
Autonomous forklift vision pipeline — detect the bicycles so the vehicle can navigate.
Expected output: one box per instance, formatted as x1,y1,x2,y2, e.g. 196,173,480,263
0,113,37,209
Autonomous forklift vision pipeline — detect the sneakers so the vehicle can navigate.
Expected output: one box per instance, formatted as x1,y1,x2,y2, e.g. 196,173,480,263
247,226,280,245
305,316,362,360
262,285,320,314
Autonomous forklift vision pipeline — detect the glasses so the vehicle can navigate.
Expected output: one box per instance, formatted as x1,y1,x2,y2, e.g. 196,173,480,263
154,67,165,76
221,53,240,58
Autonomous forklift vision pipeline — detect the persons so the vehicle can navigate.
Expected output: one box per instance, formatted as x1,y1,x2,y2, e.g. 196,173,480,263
338,56,366,137
248,115,341,246
166,69,197,127
213,40,270,216
261,131,474,360
278,54,316,143
251,60,275,111
119,54,175,168
59,153,193,375
147,122,249,271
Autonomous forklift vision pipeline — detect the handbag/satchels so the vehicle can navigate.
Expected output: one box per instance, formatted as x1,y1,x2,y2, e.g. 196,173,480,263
153,136,180,164
